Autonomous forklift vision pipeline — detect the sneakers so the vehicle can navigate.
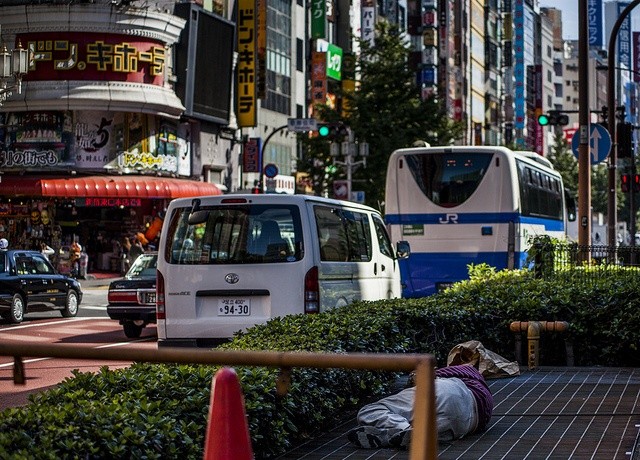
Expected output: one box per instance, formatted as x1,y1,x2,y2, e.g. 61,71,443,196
388,428,414,451
348,426,402,449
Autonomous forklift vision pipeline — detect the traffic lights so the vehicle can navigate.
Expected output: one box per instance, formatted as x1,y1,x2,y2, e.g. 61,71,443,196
538,115,568,126
318,125,330,137
621,174,629,192
634,174,640,192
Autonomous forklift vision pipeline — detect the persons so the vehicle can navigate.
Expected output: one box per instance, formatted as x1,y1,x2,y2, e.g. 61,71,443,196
38,242,55,266
71,240,81,276
120,236,130,274
346,362,495,449
131,240,139,265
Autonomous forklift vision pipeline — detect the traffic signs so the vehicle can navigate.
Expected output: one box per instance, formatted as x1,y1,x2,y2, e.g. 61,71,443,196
264,164,278,178
572,123,612,165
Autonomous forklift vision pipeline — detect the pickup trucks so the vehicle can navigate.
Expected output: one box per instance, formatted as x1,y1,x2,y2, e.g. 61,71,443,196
107,254,158,338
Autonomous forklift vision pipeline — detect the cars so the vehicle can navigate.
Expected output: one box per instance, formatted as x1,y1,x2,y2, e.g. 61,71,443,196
0,250,83,324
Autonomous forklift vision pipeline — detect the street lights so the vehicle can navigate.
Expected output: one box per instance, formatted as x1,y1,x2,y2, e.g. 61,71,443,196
595,66,640,75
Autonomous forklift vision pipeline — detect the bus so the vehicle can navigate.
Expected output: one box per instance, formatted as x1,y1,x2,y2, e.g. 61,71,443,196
384,146,576,300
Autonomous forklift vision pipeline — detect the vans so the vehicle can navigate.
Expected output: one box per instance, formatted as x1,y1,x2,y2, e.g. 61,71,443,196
156,194,411,347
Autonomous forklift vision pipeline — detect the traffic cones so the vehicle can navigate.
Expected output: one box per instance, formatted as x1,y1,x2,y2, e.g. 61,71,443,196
203,367,254,460
